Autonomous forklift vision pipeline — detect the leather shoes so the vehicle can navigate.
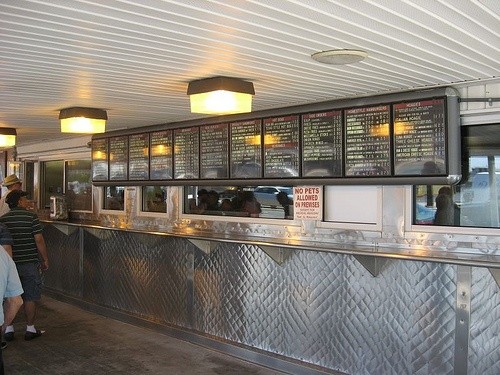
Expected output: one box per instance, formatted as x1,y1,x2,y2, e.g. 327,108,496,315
4,332,15,341
24,329,41,341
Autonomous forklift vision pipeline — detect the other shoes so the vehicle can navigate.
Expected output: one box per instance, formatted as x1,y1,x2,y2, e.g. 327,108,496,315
0,341,8,349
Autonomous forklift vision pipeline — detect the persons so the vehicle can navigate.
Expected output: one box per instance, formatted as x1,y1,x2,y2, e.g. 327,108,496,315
0,190,49,340
0,175,24,375
64,182,463,226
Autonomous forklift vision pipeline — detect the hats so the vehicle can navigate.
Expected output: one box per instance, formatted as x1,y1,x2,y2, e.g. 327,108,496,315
5,189,28,203
2,174,20,186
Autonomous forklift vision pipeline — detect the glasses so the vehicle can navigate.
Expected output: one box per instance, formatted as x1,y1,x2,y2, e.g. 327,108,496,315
15,183,22,186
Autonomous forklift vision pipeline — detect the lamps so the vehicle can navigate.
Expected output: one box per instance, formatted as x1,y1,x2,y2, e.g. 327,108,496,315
59,107,108,133
187,77,255,114
0,127,17,147
